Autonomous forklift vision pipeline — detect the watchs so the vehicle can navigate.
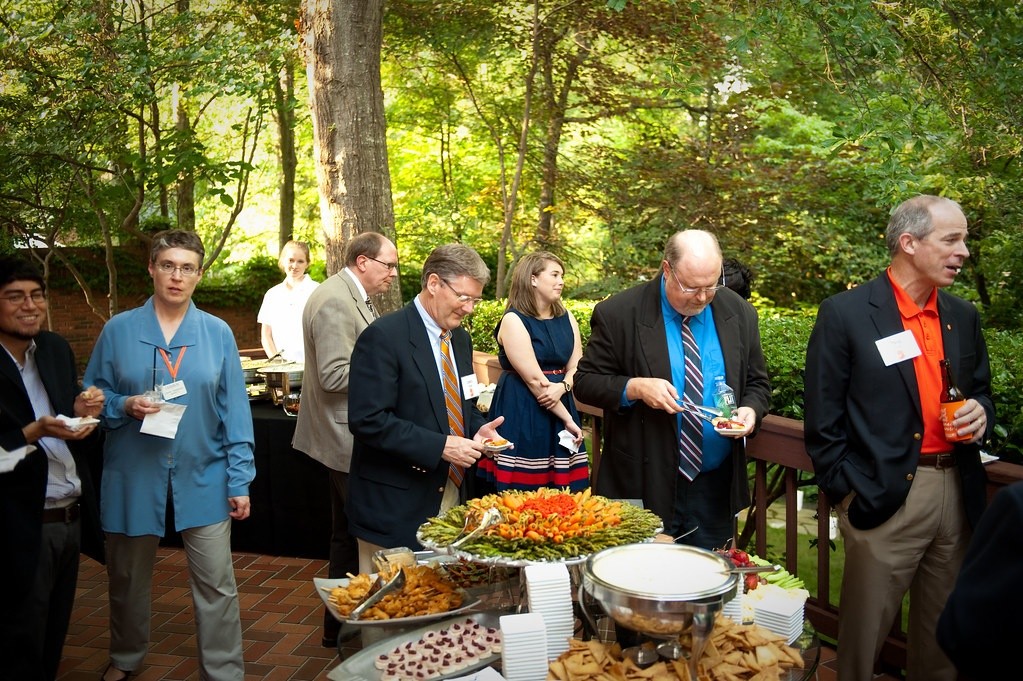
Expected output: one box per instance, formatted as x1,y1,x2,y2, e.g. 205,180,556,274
561,380,571,393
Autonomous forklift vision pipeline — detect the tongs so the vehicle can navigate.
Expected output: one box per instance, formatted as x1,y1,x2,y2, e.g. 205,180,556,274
445,508,506,557
673,396,724,423
347,568,406,621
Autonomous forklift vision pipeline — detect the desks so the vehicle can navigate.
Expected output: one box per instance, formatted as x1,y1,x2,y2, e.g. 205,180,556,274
158,396,334,561
336,613,824,681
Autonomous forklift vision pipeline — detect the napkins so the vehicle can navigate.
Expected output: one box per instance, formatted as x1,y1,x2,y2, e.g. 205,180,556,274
558,429,580,455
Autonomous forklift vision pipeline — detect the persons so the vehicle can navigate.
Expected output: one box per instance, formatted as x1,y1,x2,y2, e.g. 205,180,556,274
475,252,588,496
803,193,995,681
572,228,772,551
257,241,320,361
292,233,397,649
719,258,751,299
81,228,257,681
346,245,513,648
0,256,104,681
932,482,1022,681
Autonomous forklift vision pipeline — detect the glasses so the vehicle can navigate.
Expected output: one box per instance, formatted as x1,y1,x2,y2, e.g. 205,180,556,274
0,292,45,305
364,254,397,271
441,278,483,306
662,260,726,296
152,261,200,274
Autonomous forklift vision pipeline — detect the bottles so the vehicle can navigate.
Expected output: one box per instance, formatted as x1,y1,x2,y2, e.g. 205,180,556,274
938,359,973,443
712,374,739,419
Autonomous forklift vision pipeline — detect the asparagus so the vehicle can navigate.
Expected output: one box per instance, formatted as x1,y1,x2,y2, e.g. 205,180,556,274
418,498,663,560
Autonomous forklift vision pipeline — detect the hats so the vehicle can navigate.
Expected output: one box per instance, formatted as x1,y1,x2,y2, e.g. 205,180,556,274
0,252,46,290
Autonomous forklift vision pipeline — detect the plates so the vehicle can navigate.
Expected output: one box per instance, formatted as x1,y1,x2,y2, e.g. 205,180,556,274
481,442,514,451
498,563,575,681
326,610,513,681
714,424,747,436
721,573,806,646
65,417,101,431
312,564,483,627
416,515,664,564
980,450,1000,464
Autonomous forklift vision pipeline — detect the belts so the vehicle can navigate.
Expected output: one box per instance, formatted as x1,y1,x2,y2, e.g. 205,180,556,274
918,455,957,470
44,504,81,524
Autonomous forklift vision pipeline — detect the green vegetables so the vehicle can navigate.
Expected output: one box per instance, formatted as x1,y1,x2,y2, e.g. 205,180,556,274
451,562,516,584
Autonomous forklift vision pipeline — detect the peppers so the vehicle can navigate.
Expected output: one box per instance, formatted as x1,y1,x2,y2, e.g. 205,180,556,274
463,486,628,542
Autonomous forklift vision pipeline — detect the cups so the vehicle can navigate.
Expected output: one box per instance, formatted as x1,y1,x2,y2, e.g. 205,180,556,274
283,394,301,417
143,366,164,408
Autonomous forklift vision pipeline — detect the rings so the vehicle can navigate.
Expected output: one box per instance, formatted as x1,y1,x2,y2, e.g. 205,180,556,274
581,436,584,439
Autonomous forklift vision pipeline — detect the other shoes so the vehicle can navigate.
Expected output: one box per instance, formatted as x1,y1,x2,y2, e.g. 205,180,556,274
99,664,129,681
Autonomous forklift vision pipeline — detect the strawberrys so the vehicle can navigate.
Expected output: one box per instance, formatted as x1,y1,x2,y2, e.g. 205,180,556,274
715,548,761,590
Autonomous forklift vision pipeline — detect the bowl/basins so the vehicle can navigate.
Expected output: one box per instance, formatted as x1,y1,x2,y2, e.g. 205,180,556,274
583,542,739,639
239,356,305,388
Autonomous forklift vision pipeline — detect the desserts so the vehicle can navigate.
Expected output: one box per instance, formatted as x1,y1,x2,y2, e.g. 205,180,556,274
375,618,502,681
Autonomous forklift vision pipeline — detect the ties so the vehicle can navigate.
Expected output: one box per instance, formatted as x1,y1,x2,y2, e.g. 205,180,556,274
678,315,704,482
439,330,466,488
